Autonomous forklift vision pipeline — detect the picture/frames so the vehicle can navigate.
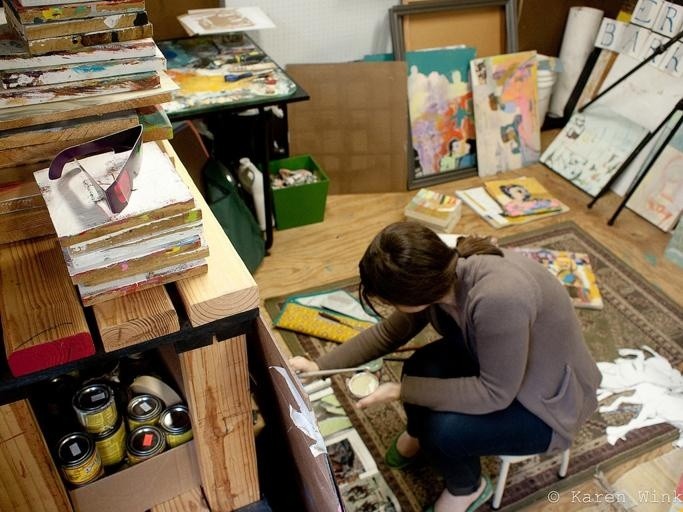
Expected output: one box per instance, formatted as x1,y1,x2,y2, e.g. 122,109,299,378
388,0,519,191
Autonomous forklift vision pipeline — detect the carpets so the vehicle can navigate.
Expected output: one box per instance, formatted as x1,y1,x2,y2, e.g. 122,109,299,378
258,218,682,512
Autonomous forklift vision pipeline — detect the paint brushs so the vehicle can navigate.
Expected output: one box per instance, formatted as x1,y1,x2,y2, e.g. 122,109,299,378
297,367,365,376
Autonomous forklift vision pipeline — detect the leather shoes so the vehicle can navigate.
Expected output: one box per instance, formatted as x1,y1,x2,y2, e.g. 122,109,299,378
423,474,494,512
385,429,423,468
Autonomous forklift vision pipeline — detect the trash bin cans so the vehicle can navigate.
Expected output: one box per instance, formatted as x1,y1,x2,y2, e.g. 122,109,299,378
259,153,330,231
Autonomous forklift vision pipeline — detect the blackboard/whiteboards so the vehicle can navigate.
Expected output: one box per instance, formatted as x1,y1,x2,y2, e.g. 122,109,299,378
258,306,345,512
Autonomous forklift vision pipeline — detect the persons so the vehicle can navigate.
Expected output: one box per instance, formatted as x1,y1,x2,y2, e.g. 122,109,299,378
286,221,603,512
